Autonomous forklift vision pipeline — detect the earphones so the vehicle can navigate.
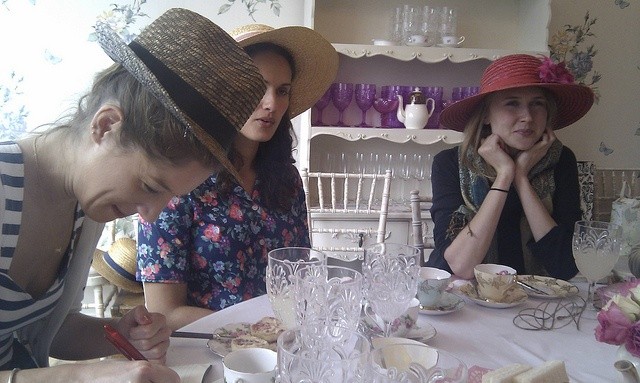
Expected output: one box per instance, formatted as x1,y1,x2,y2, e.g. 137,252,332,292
512,282,596,330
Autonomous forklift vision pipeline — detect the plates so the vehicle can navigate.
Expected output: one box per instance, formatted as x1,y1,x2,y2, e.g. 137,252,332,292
414,291,465,316
206,322,277,357
437,43,464,48
366,336,439,371
512,274,580,298
357,318,437,344
405,42,434,47
464,278,529,309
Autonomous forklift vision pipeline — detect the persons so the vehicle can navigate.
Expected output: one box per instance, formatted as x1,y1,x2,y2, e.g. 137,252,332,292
430,53,581,281
0,8,224,383
135,23,313,333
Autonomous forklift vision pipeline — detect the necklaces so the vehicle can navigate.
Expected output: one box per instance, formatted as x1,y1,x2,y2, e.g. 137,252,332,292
33,134,74,257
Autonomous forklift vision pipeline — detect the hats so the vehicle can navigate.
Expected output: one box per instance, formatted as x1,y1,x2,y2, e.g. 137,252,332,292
94,7,266,191
436,53,594,132
91,237,146,293
226,22,338,120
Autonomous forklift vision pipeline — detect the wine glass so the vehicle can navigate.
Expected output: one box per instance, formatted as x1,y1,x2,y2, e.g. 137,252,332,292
293,265,364,324
275,324,371,383
354,82,376,128
330,81,354,128
572,220,623,320
372,97,399,129
461,85,481,101
364,243,420,342
366,343,466,383
266,246,327,332
316,150,433,208
451,87,463,102
311,86,332,127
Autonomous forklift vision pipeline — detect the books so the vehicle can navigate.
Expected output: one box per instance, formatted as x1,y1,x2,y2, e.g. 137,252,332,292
169,364,212,383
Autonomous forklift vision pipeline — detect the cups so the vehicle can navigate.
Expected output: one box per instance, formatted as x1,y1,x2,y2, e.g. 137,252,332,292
473,263,519,300
440,6,458,43
423,5,441,47
222,348,277,383
381,85,387,128
442,36,465,45
391,7,403,46
408,34,429,44
364,297,420,337
386,84,408,128
402,4,423,46
426,86,443,129
417,266,455,307
408,85,427,98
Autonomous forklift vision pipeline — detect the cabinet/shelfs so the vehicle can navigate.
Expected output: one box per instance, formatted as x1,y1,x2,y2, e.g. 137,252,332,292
312,1,551,222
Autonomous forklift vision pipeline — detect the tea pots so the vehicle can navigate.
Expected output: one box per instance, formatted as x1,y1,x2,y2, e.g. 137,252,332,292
396,86,436,130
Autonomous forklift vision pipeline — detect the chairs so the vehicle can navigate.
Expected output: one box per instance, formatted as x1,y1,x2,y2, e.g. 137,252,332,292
411,190,434,265
301,168,392,259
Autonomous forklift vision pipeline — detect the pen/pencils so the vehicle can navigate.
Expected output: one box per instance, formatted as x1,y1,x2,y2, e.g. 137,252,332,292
103,324,147,361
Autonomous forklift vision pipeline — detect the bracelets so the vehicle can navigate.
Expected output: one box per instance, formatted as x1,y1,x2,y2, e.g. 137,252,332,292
487,188,510,193
7,368,20,383
